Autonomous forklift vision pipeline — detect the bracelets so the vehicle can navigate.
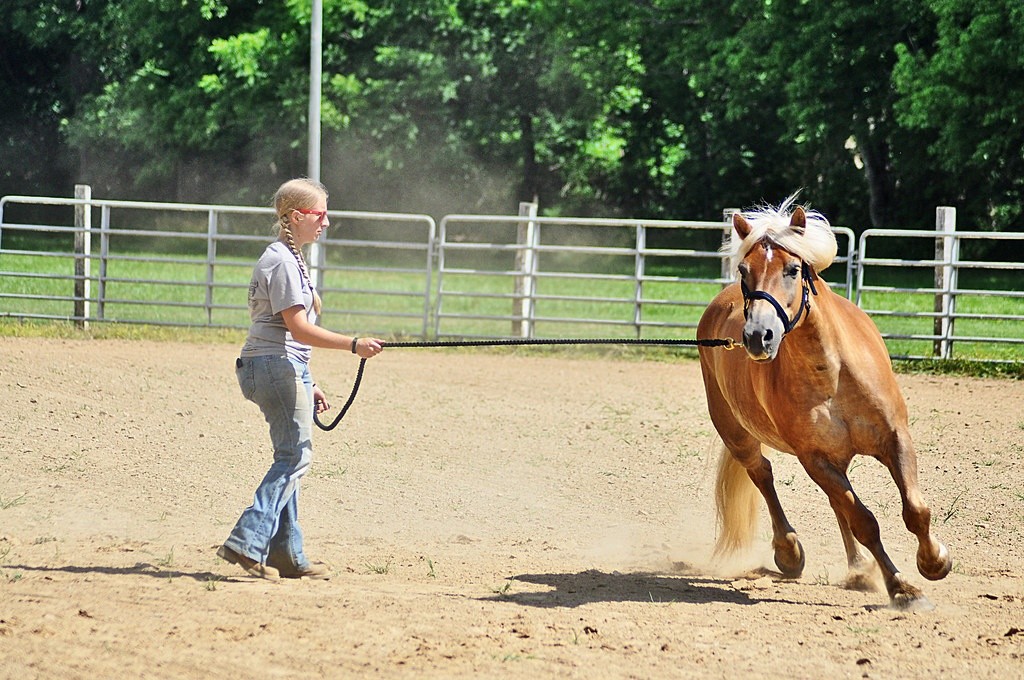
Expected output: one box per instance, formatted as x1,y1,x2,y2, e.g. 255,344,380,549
351,337,359,354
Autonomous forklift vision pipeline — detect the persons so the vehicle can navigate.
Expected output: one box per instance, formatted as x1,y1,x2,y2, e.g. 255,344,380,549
217,179,386,582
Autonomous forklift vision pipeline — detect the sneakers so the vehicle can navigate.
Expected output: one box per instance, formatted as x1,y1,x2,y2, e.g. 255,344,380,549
216,545,279,581
265,558,331,581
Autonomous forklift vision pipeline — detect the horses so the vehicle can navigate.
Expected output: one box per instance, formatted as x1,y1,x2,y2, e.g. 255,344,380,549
695,187,952,606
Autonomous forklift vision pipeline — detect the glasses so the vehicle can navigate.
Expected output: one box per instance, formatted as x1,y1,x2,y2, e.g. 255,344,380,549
296,207,326,222
